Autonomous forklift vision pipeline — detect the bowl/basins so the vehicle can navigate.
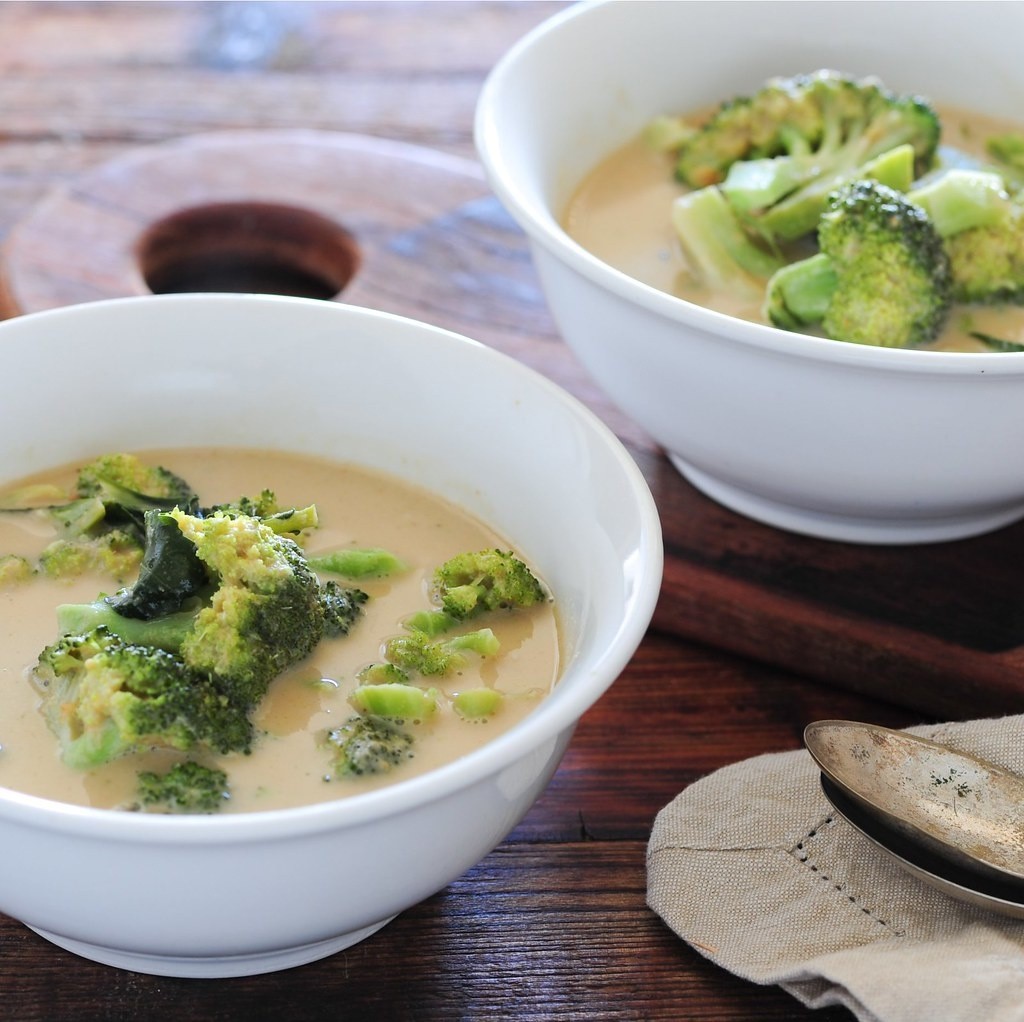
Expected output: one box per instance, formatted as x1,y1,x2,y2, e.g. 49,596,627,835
471,1,1024,546
0,294,665,979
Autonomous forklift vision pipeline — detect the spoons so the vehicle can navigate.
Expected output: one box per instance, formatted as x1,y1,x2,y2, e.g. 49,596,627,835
801,714,1024,922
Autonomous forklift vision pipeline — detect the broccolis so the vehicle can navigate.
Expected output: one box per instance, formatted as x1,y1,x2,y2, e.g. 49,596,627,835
645,67,1024,353
1,454,564,824
